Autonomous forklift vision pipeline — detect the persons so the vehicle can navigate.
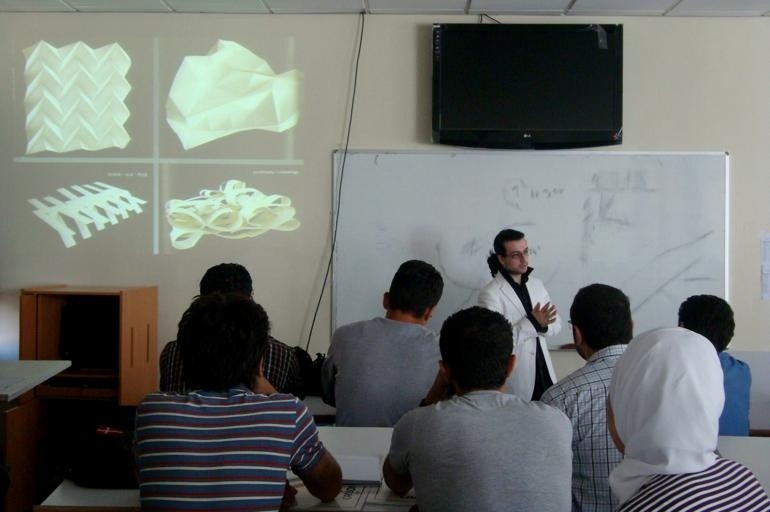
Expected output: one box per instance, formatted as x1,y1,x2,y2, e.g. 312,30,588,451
477,226,564,405
602,325,770,512
157,261,316,406
381,301,573,511
538,282,648,512
321,259,448,424
676,291,752,435
135,291,343,512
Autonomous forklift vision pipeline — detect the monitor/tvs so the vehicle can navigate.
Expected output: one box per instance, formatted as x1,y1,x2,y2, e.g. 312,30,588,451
431,21,624,150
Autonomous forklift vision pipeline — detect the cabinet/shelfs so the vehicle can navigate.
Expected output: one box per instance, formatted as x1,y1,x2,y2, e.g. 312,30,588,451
18,281,159,407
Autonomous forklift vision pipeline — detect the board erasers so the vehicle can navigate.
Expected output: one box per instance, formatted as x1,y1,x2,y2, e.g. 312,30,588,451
560,344,576,350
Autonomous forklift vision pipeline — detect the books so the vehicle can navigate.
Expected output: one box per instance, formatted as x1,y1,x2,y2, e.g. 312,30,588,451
285,454,419,512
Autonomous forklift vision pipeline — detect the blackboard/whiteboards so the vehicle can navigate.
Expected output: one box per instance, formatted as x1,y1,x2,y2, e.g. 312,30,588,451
331,150,730,351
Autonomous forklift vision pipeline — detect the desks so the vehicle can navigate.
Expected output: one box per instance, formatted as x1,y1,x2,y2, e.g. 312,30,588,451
39,477,141,511
0,360,73,510
298,396,338,416
279,426,420,510
714,434,770,501
